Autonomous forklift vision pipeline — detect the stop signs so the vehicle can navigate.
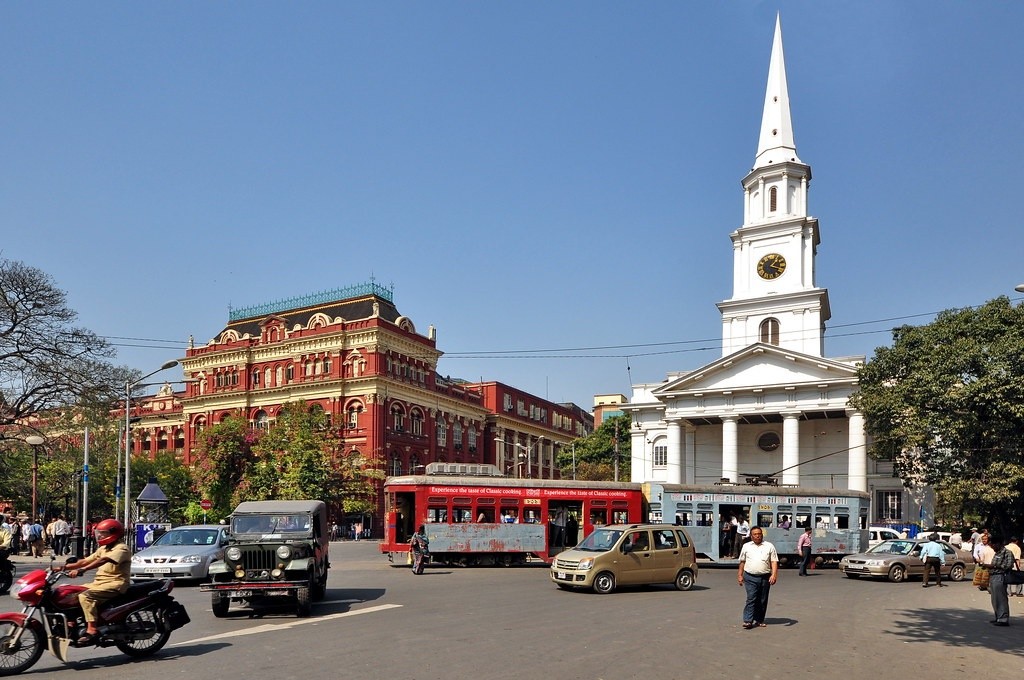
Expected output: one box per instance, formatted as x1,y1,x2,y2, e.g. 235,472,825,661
201,499,213,510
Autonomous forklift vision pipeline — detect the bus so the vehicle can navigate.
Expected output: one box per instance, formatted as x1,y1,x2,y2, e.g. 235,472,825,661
379,460,641,567
643,435,893,569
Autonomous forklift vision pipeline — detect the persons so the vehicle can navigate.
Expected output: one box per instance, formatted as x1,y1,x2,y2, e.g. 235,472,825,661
395,497,625,551
44,519,132,644
978,535,1015,626
409,525,430,576
719,514,824,577
279,520,361,543
738,526,779,629
897,528,1024,596
0,515,124,583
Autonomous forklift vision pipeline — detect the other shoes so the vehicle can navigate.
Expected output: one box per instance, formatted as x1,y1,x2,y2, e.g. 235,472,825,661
1016,594,1023,597
78,633,101,645
799,573,806,577
922,583,929,587
994,623,1009,627
990,620,998,623
755,622,767,627
744,623,752,628
34,556,38,558
936,584,942,587
38,554,43,558
732,556,737,558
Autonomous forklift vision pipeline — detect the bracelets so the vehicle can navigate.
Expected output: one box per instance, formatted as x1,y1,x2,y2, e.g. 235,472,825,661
993,566,996,568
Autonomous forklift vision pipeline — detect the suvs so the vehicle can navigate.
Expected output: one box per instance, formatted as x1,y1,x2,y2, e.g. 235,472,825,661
200,500,331,619
550,523,698,594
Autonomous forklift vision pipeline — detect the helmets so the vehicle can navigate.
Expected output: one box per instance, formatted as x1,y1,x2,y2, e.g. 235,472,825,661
95,520,124,546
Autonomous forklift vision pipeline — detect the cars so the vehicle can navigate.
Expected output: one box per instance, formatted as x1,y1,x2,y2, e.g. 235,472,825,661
869,526,973,553
838,539,975,583
130,525,230,583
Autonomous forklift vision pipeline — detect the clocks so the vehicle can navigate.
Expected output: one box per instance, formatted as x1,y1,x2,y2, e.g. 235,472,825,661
757,255,786,280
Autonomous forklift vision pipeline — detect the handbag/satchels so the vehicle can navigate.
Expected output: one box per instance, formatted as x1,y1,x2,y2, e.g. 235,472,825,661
1008,570,1024,585
28,534,37,541
973,568,990,588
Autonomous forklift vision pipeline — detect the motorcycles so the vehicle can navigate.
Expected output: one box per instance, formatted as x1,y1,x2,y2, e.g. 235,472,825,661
0,547,16,596
0,556,190,676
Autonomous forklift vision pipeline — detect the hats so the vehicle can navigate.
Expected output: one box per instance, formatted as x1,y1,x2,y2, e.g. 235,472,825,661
970,526,978,531
903,528,910,532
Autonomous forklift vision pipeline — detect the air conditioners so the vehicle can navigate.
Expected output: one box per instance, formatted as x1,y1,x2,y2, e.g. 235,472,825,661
470,446,476,452
396,425,404,430
455,444,463,449
349,422,356,428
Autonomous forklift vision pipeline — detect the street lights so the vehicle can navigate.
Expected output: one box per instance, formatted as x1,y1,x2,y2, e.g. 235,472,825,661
124,360,201,546
494,435,546,479
115,417,142,520
555,441,575,480
26,437,44,524
